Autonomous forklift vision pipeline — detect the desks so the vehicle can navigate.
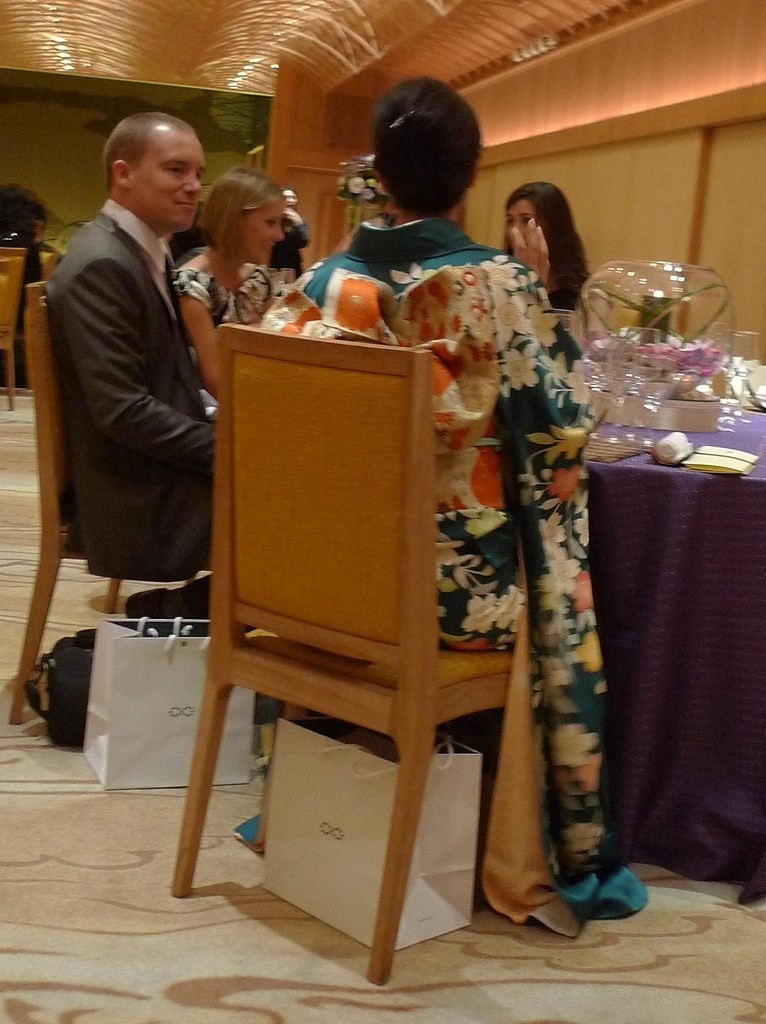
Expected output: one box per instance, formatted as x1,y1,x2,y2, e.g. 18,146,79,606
587,401,766,905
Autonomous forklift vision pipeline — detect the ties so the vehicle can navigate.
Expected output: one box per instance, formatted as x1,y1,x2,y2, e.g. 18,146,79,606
164,253,192,363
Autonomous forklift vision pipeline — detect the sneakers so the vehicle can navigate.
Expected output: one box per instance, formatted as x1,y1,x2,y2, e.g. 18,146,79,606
264,716,484,952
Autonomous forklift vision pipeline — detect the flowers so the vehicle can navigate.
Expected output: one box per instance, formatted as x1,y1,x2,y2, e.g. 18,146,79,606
334,143,398,230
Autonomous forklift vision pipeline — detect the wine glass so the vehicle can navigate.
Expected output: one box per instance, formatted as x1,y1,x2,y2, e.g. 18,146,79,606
717,333,741,433
732,330,761,426
582,345,680,447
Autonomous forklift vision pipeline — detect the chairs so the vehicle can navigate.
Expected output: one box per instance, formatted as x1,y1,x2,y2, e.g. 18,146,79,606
0,239,518,992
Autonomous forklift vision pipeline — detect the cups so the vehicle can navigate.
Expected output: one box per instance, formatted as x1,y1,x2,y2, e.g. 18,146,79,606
268,267,298,295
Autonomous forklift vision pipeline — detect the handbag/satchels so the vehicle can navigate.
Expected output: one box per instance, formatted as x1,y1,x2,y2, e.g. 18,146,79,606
84,618,256,790
25,627,96,748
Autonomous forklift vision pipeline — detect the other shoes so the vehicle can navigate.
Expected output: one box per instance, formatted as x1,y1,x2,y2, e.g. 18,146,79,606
126,588,166,618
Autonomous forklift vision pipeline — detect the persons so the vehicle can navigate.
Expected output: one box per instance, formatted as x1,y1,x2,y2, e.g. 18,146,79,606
172,166,287,401
234,77,648,938
41,111,215,620
504,181,589,310
0,184,43,283
270,188,311,278
